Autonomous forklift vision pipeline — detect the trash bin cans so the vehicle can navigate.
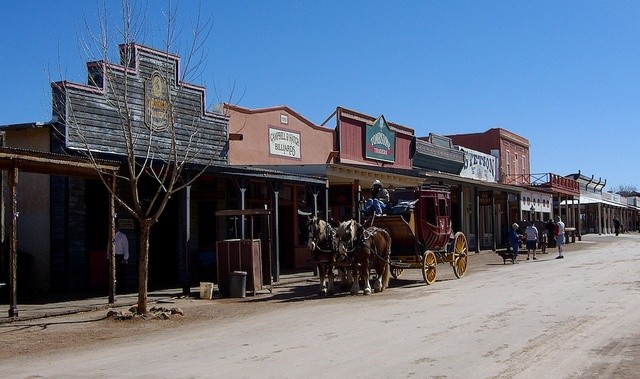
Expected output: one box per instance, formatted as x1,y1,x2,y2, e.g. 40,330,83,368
227,271,248,298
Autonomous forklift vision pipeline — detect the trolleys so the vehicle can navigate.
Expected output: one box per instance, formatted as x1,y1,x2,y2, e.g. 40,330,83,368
494,240,517,264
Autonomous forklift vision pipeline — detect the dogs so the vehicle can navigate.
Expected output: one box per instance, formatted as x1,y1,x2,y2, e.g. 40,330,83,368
497,251,517,264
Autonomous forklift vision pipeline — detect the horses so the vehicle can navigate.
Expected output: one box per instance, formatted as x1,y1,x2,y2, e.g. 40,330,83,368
334,219,392,295
305,215,352,296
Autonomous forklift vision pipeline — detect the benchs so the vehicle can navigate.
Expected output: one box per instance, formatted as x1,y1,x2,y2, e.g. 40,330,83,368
383,190,415,214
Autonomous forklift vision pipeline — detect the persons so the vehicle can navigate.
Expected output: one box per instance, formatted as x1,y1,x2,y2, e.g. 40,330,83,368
612,217,621,237
106,220,131,293
509,222,523,264
360,179,390,216
524,221,540,261
554,216,566,260
545,219,555,248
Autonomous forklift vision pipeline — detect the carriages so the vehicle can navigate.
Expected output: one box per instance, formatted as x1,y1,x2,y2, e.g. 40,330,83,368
306,180,470,295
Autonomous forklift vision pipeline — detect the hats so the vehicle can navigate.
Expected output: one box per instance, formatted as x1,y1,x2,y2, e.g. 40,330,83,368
373,180,383,188
512,223,519,228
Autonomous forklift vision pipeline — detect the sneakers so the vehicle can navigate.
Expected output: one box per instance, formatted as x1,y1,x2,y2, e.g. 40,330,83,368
526,258,529,260
555,256,563,259
533,258,536,260
